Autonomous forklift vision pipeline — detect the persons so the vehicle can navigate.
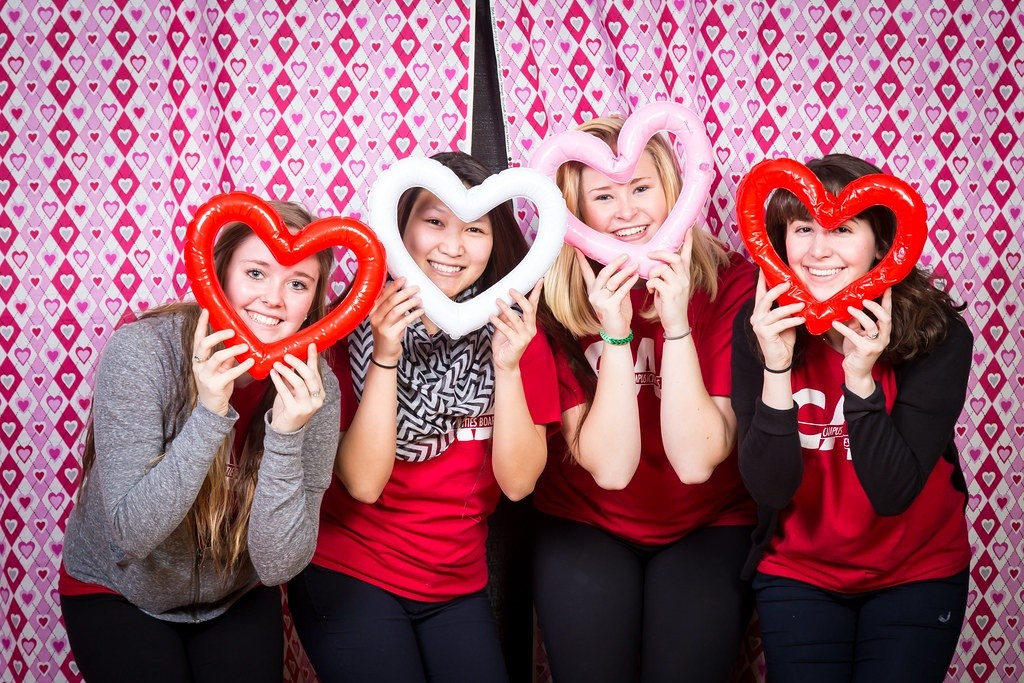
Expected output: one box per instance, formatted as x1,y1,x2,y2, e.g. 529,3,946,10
534,114,775,683
57,201,341,683
286,149,563,683
731,153,974,683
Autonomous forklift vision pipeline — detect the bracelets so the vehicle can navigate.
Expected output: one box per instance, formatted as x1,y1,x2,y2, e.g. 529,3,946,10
763,362,792,373
600,328,633,345
662,326,692,340
369,353,401,369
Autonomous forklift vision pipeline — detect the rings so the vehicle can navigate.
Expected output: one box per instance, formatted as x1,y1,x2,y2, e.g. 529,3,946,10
866,330,879,340
604,284,614,293
310,388,322,397
194,356,205,362
